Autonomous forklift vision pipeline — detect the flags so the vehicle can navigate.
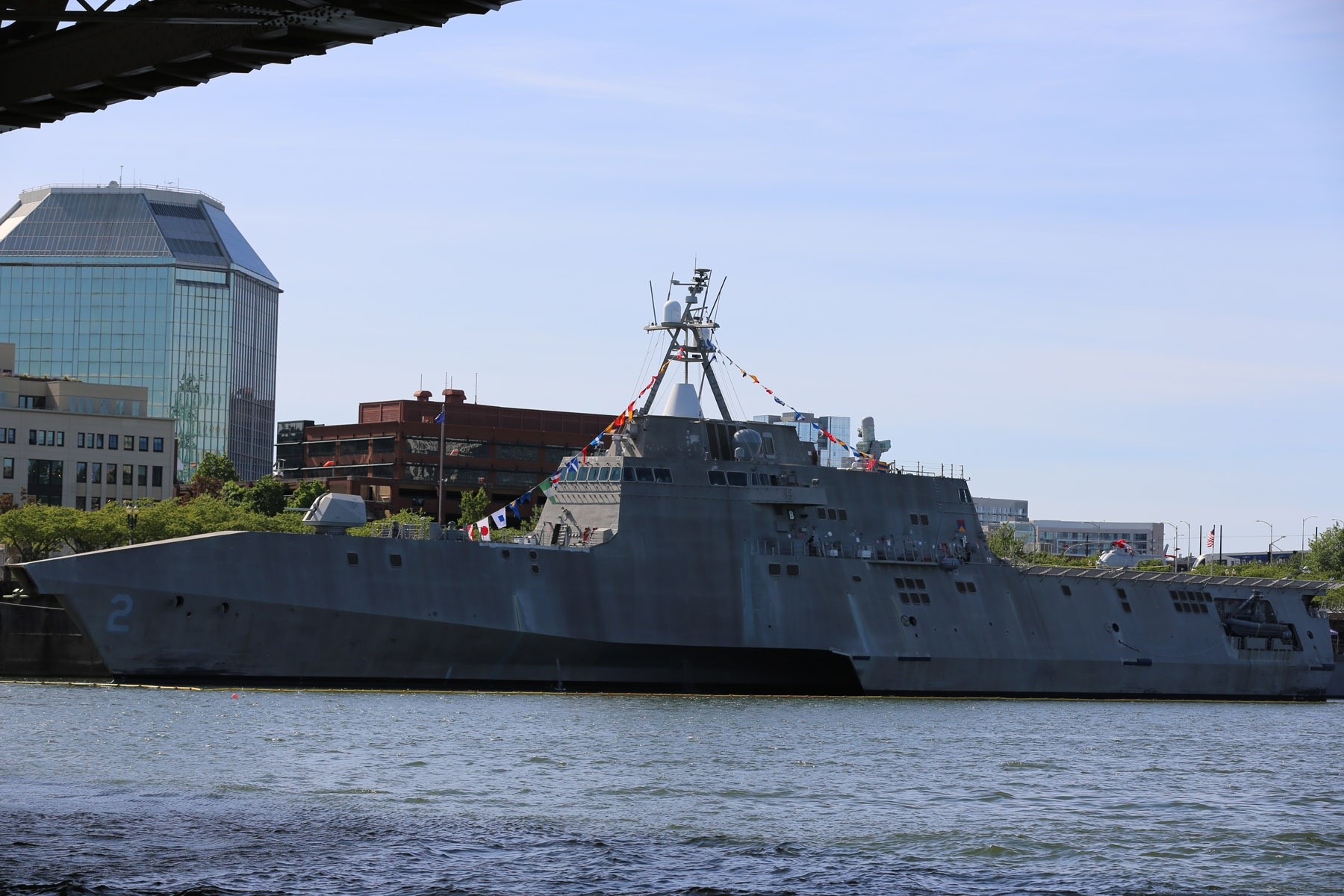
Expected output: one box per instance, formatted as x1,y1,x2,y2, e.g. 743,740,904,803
627,401,635,421
749,375,759,384
836,438,849,452
795,412,805,420
774,396,785,406
491,507,506,529
539,479,556,504
823,430,836,444
812,423,824,437
590,432,603,447
582,447,589,463
1206,529,1214,547
508,501,520,520
550,468,562,490
760,384,773,394
605,423,613,433
520,491,531,506
434,411,446,424
861,451,873,461
566,457,578,472
850,447,862,462
459,525,473,541
614,411,625,426
675,347,683,359
476,516,491,542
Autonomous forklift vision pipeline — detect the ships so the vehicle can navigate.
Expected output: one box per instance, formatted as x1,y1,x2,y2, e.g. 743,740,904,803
3,265,1343,700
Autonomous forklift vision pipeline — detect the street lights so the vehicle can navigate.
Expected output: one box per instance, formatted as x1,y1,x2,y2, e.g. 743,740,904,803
1254,520,1273,562
1160,522,1180,573
1180,520,1191,571
1173,534,1185,567
1301,515,1318,560
1081,521,1100,550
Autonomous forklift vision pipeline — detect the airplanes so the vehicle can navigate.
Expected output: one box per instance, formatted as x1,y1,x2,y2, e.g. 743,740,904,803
1096,544,1169,569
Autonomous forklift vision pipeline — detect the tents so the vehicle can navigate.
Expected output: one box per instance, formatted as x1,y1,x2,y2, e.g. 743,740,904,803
1330,629,1339,654
